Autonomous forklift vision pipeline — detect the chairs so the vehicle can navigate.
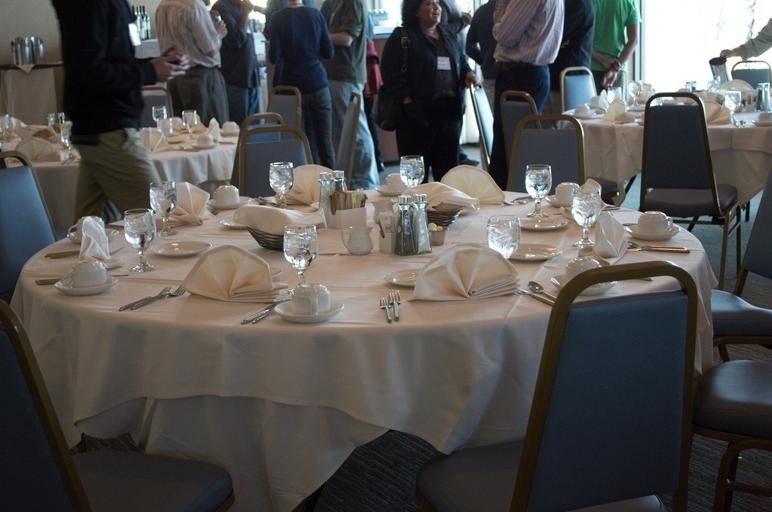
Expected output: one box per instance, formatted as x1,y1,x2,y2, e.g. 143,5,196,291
0,150,57,301
1,303,235,512
0,63,362,238
465,56,772,291
694,168,772,511
415,260,699,512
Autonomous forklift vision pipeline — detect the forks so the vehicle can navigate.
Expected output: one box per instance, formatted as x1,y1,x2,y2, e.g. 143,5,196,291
118,283,187,313
376,284,404,323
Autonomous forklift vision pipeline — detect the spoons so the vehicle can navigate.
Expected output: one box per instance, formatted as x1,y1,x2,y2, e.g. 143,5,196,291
625,243,689,253
497,193,531,208
528,280,558,306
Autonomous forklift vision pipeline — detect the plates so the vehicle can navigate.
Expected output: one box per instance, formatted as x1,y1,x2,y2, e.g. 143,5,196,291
206,195,251,212
628,224,680,242
384,267,423,289
54,228,120,297
151,240,209,257
219,217,248,231
274,298,345,324
508,241,562,264
550,275,619,297
373,186,405,198
517,216,570,232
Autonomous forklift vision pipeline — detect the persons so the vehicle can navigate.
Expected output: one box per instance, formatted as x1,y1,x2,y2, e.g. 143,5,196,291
49,0,164,233
715,16,772,60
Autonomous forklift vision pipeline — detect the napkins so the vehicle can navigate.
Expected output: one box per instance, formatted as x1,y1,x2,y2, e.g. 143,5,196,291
407,243,521,302
401,164,505,214
78,218,111,262
183,246,290,303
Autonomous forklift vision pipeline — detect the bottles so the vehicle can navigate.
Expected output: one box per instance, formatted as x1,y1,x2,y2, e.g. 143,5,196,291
130,4,153,41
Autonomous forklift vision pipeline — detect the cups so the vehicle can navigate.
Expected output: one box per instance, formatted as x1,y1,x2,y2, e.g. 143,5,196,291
0,104,239,164
637,210,675,233
210,8,223,26
557,183,582,204
574,77,772,128
341,225,375,255
66,216,107,241
291,280,332,316
213,186,241,207
384,173,404,186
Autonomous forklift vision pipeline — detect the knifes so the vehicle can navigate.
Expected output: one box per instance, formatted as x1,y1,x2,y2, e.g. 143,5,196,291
240,288,294,325
515,287,555,308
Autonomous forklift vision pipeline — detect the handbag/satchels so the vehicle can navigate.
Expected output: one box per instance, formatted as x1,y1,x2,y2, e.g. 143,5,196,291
376,27,410,130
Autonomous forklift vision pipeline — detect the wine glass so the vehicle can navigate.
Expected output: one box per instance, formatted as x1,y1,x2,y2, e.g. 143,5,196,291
122,209,157,274
281,224,319,281
485,214,525,262
570,185,602,248
522,163,554,219
267,162,297,208
400,156,425,188
148,179,182,238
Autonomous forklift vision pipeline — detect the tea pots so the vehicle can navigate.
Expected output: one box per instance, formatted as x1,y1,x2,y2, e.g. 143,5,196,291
10,34,45,66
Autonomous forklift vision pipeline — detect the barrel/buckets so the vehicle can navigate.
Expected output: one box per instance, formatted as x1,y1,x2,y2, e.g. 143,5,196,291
10,35,44,65
